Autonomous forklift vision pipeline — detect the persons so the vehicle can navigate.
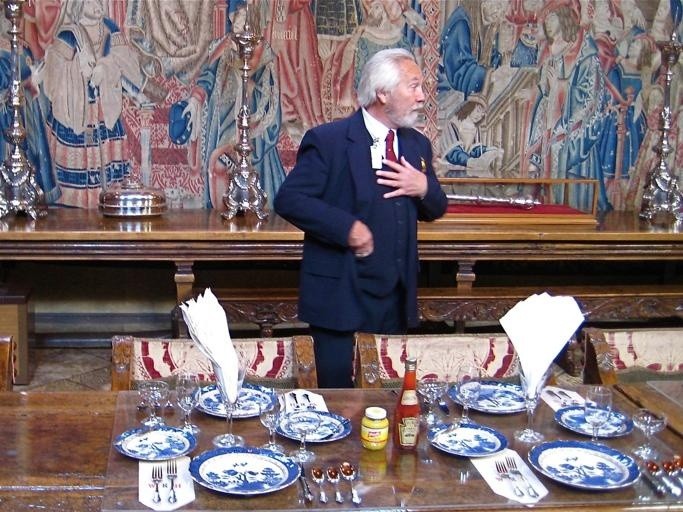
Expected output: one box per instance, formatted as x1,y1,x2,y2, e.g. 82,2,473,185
273,46,447,387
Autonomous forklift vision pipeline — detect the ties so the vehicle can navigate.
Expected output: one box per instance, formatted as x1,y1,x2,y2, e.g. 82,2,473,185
385,130,398,162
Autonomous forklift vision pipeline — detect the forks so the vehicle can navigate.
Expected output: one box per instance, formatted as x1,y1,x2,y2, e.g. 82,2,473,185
495,459,524,498
505,457,539,498
151,465,162,503
166,458,181,505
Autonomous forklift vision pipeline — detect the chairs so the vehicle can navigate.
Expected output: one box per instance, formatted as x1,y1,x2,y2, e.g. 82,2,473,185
0,335,16,393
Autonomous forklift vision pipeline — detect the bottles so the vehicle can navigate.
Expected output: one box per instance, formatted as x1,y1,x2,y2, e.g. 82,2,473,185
390,448,417,509
357,449,389,485
393,360,422,453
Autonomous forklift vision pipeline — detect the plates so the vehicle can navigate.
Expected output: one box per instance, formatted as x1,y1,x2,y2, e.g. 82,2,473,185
110,425,198,461
552,403,634,441
188,446,303,498
272,409,353,444
526,441,641,491
190,380,276,420
448,377,539,416
427,422,509,460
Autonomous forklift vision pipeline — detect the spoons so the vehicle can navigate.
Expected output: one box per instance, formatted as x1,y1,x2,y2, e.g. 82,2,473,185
339,462,361,504
311,465,329,503
638,457,683,505
324,466,345,503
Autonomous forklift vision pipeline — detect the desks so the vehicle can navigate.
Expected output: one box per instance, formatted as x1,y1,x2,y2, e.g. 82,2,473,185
0,210,683,338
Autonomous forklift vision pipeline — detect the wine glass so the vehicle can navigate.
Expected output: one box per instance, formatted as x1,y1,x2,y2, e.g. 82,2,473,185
174,373,201,435
512,372,547,441
630,407,668,461
417,375,447,425
583,385,612,444
259,390,286,454
214,369,244,447
288,404,319,463
136,379,170,426
449,375,480,424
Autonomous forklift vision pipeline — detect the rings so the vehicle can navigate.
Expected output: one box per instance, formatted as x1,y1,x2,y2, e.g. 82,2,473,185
351,233,359,242
